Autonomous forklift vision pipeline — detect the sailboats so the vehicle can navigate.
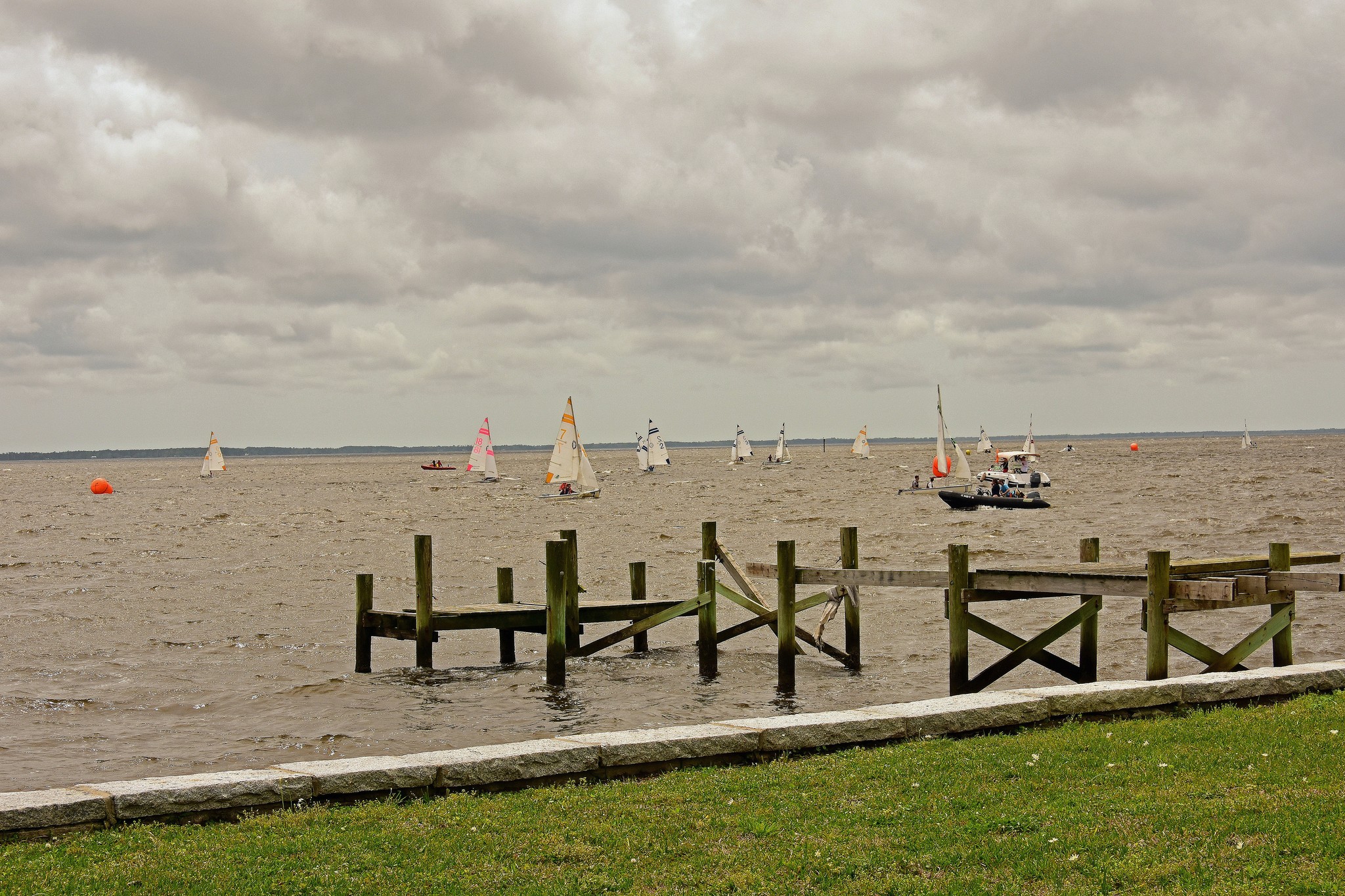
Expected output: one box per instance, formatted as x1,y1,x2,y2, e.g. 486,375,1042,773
199,431,227,479
731,424,754,465
634,418,672,476
1010,413,1037,464
540,396,602,501
898,383,974,497
976,425,994,454
1241,418,1258,450
851,425,877,460
465,417,503,483
761,421,791,467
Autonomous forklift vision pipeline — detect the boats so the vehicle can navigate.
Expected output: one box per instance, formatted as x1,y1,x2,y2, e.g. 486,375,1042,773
420,464,457,471
1063,447,1075,451
937,486,1052,509
975,451,1053,488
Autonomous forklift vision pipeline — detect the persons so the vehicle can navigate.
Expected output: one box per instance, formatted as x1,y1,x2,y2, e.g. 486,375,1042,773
559,482,573,495
926,478,936,488
767,454,773,462
776,458,781,463
985,449,991,453
913,475,921,489
648,465,654,471
988,465,994,471
1014,455,1021,462
1067,444,1072,450
998,457,1008,473
1253,442,1257,446
430,460,441,467
991,478,1010,497
738,457,743,461
1020,456,1028,473
208,471,212,477
